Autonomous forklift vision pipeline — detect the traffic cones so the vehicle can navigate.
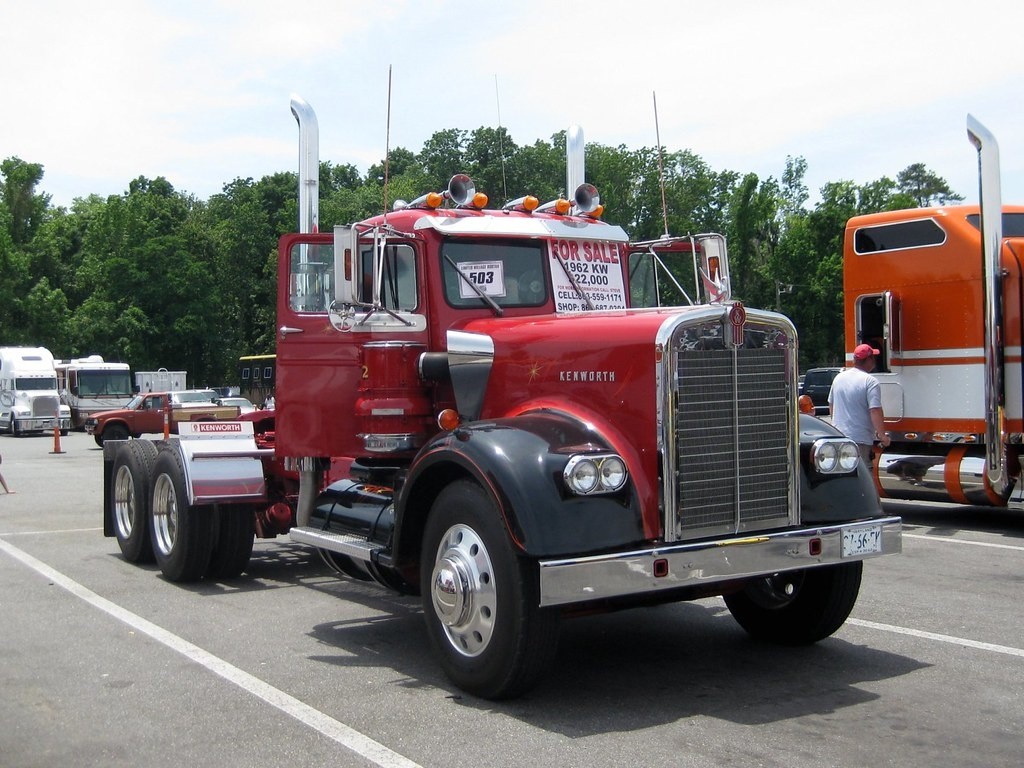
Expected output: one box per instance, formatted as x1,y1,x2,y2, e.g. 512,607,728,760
48,427,67,454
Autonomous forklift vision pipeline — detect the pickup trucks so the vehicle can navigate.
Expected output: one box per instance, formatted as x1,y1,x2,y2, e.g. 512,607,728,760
87,391,241,450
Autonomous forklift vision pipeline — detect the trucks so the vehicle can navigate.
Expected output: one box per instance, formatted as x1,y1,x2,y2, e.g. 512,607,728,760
54,357,131,431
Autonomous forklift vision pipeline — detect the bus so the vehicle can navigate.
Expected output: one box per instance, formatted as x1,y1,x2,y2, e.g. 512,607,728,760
208,387,240,399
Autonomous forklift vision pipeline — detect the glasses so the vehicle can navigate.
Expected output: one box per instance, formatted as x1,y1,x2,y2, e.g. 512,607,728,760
866,356,877,361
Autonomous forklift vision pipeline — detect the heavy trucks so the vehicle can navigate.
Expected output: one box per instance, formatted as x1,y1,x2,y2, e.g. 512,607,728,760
99,176,904,691
0,346,71,435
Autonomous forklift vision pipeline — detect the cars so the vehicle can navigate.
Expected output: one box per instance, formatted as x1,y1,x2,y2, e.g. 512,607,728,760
795,374,808,396
215,397,261,415
187,389,222,403
802,368,846,415
163,391,217,408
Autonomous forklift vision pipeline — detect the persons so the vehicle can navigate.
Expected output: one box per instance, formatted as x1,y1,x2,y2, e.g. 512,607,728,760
827,345,891,478
265,394,275,408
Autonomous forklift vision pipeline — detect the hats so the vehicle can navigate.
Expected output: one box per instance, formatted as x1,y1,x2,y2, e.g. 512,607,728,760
854,344,880,360
